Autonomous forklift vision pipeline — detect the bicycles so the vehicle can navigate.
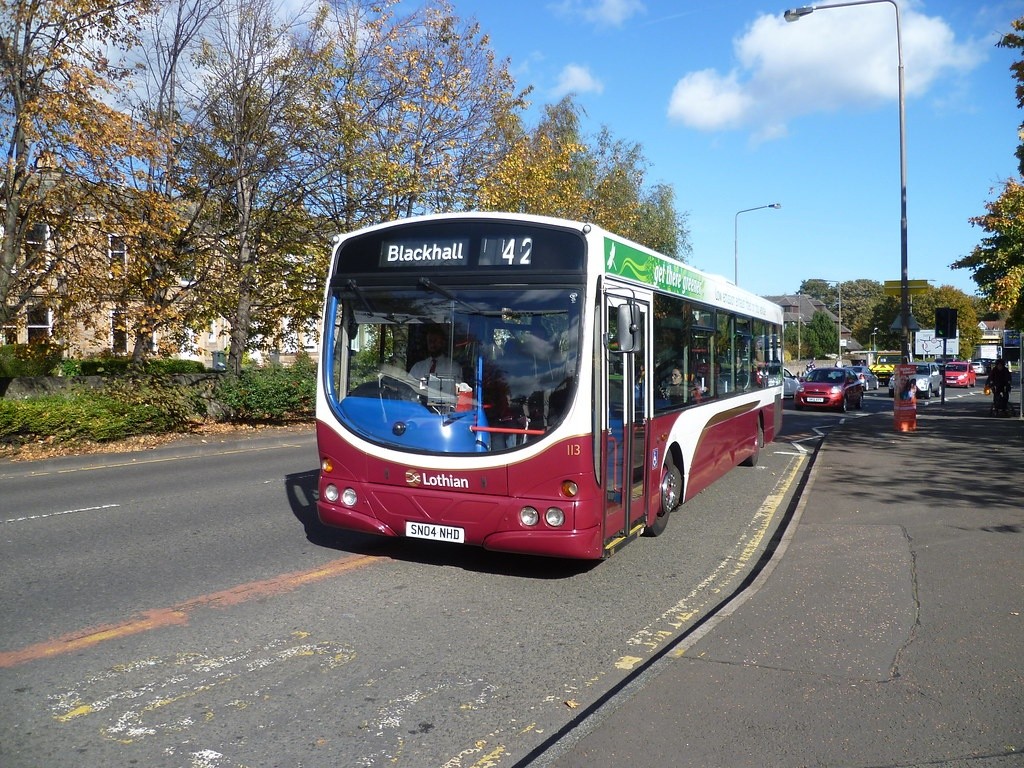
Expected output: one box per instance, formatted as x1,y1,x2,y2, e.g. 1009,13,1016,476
988,380,1016,418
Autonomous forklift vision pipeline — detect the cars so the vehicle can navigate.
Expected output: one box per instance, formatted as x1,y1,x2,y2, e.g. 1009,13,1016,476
888,362,942,399
970,360,988,375
793,367,865,414
933,358,958,378
945,362,977,389
783,367,799,399
844,365,880,390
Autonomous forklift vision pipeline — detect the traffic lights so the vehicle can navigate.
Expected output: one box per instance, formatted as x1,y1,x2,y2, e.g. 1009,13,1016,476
934,307,951,340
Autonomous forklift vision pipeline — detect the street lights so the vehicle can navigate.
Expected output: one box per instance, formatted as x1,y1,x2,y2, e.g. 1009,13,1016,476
869,333,877,351
734,203,782,287
873,327,879,352
783,0,912,364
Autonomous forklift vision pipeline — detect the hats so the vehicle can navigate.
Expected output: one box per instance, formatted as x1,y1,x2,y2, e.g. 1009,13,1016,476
994,359,1006,365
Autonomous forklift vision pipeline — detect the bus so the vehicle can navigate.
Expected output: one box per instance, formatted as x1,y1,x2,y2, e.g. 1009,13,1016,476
312,210,785,563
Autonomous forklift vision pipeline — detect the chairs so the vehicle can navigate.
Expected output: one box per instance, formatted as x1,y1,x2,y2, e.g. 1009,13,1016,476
696,363,721,399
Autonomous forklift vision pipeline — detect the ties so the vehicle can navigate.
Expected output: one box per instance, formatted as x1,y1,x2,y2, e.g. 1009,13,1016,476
430,359,437,373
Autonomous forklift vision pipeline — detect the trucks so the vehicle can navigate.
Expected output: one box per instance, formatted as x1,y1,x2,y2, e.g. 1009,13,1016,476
868,350,902,386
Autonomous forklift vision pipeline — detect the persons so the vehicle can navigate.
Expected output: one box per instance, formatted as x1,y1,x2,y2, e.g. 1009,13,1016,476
984,359,1012,411
803,357,816,377
406,328,462,403
898,375,916,400
857,359,865,366
634,337,763,417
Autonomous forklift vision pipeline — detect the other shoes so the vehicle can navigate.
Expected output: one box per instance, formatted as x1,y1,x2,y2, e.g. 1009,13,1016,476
1003,408,1011,417
993,408,1001,416
